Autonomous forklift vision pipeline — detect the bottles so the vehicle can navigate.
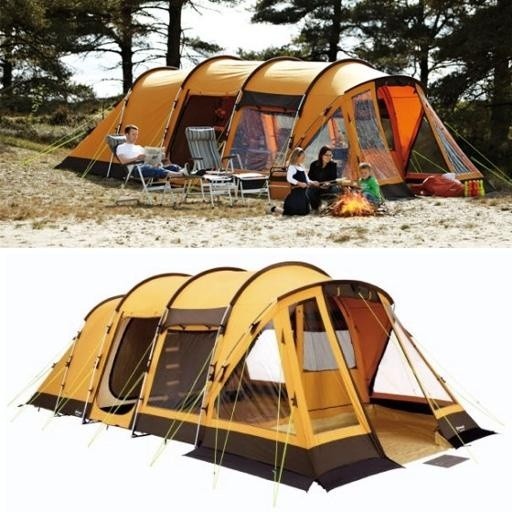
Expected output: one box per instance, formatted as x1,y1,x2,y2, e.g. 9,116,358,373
464,179,485,197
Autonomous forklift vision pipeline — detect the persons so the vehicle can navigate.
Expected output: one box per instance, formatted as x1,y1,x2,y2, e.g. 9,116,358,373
282,146,322,217
349,162,383,215
115,124,206,182
307,145,339,215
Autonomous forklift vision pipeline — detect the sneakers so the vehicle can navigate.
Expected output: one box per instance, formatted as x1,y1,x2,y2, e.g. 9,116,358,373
182,163,190,176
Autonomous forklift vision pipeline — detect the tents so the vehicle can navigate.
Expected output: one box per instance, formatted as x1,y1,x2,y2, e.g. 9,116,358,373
29,262,497,491
53,56,496,210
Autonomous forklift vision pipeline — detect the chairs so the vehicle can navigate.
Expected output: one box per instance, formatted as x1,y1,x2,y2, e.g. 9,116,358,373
99,134,232,209
185,125,271,203
